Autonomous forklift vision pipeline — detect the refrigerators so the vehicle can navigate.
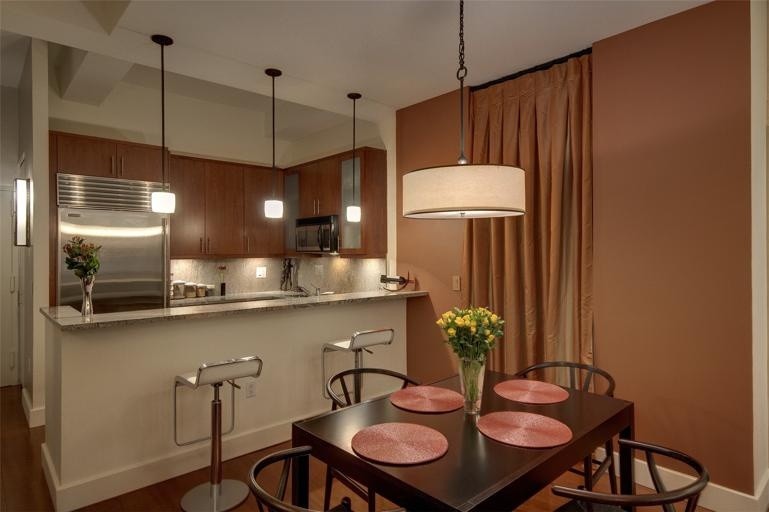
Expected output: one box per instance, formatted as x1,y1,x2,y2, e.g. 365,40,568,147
56,206,171,313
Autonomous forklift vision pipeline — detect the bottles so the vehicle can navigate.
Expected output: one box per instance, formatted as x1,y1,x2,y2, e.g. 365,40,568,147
380,275,415,285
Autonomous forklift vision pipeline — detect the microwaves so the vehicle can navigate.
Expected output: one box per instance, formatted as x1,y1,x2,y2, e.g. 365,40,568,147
295,216,338,252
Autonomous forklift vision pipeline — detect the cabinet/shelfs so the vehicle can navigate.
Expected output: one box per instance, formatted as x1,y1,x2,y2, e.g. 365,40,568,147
298,155,339,219
50,134,170,182
242,165,286,260
168,152,244,259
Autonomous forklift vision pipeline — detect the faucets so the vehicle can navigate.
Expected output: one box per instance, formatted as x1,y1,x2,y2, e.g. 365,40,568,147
300,283,320,297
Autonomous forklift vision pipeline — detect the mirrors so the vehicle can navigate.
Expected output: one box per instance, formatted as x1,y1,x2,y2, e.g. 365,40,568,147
340,148,365,258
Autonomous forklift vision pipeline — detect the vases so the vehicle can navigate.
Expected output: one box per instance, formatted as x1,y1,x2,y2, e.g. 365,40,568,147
79,278,96,316
455,356,487,417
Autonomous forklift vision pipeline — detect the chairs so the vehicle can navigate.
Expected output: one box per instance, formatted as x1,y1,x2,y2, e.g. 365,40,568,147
320,328,394,404
246,444,352,512
551,438,711,512
325,367,421,409
513,361,616,491
172,355,262,512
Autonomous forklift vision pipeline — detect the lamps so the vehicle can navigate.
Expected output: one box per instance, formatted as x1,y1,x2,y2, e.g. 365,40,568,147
262,66,287,221
343,92,363,221
13,176,31,249
402,14,526,221
147,33,178,216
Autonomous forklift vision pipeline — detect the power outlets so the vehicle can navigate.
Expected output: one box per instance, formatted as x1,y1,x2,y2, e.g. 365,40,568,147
255,266,267,280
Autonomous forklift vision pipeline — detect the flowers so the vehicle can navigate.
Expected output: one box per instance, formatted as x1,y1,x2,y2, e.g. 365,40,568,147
63,238,104,277
434,303,503,360
